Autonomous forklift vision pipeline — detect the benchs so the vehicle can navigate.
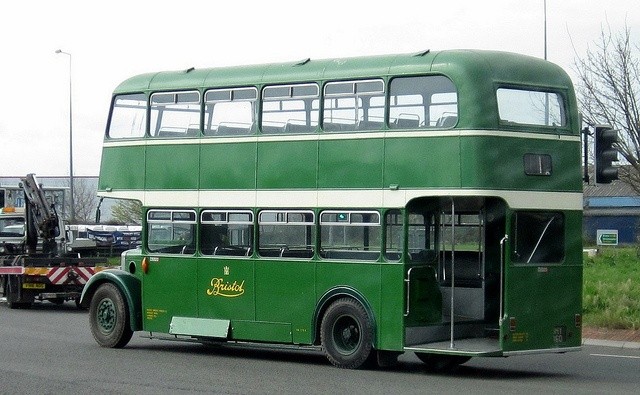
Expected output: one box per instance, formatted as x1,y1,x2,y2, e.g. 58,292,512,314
285,118,313,132
216,122,250,134
359,116,397,128
396,113,420,127
185,124,215,136
438,111,457,127
157,127,185,136
324,117,359,131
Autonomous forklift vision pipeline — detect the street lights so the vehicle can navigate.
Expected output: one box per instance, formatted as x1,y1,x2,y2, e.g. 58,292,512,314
55,48,75,223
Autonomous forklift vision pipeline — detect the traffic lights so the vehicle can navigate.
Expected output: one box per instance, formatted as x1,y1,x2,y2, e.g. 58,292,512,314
601,169,619,181
601,129,621,143
602,150,621,164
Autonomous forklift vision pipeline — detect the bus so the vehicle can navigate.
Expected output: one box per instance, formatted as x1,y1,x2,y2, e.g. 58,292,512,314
78,48,583,369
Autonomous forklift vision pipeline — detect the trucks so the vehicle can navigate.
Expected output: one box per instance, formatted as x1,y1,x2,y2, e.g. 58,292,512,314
0,208,142,255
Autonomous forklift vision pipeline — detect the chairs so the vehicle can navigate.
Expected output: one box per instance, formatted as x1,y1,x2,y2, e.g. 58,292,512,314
211,246,222,255
179,245,189,254
277,246,288,258
244,247,252,256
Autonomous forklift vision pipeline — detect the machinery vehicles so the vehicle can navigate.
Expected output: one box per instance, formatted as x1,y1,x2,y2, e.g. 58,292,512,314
0,173,118,309
